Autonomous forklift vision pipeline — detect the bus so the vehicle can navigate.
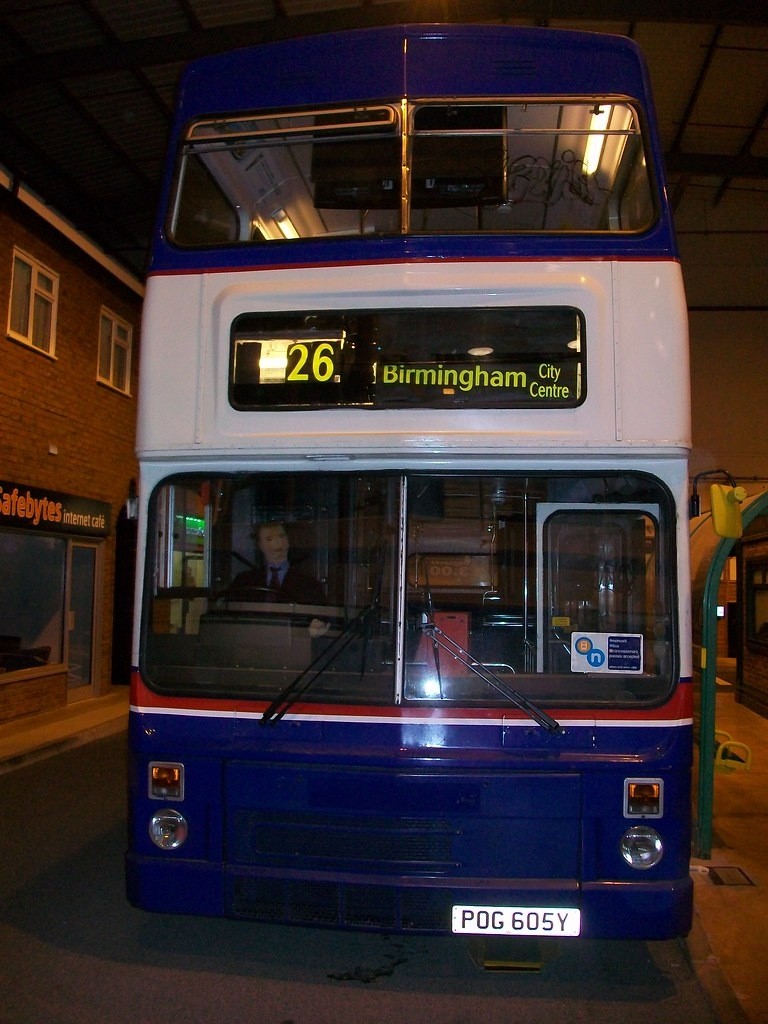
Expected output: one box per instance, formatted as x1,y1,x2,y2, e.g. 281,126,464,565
122,23,750,938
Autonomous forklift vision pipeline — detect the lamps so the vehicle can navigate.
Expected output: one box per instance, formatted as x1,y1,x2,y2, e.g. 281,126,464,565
271,207,299,238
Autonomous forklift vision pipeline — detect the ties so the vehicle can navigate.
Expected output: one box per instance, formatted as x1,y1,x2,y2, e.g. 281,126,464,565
270,566,281,587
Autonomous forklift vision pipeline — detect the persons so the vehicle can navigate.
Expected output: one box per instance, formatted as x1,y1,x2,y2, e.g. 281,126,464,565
217,519,331,639
